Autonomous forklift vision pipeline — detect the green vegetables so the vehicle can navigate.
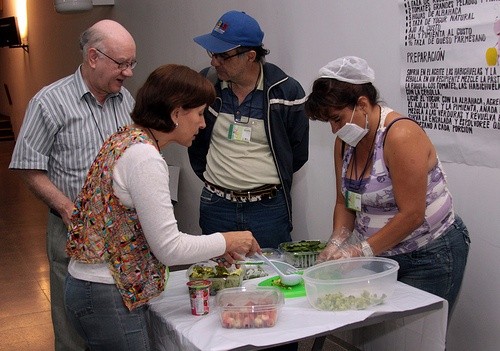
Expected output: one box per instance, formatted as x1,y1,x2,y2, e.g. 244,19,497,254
191,263,241,295
281,240,327,266
317,289,385,311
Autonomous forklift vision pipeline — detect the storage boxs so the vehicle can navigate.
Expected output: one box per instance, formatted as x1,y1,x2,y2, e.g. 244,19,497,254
186,263,242,296
280,240,328,267
215,288,282,328
303,257,400,310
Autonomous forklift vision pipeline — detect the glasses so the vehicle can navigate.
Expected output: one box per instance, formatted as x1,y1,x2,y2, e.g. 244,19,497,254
206,47,255,63
95,47,138,71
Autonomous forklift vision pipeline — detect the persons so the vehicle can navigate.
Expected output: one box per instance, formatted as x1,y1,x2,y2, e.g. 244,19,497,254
305,57,471,331
60,63,263,351
185,9,314,245
8,19,137,351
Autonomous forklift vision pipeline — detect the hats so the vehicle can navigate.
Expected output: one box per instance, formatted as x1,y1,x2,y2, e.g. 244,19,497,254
193,11,264,53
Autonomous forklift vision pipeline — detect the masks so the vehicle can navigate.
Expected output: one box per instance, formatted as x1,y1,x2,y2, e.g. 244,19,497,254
333,106,370,148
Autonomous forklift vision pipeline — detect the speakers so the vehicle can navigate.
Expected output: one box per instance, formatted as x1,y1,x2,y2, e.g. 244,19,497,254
0,16,22,48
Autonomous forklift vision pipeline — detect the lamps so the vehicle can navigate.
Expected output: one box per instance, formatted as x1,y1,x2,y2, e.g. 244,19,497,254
0,16,29,55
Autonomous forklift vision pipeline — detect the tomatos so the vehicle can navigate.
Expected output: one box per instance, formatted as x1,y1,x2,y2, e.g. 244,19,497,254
222,297,276,328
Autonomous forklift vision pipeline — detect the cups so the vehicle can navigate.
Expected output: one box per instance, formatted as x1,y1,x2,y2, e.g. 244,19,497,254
186,279,212,316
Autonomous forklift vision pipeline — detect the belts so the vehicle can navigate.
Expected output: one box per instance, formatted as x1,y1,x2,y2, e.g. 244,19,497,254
47,205,63,220
204,180,284,203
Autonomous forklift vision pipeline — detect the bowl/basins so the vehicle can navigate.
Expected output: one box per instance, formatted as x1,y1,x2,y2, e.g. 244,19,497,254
214,286,283,330
302,257,400,312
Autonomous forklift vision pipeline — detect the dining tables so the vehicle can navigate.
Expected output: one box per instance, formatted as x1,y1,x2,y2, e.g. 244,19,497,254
141,265,450,351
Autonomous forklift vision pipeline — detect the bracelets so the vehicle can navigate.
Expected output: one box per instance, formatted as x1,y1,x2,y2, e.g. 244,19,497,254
329,238,341,247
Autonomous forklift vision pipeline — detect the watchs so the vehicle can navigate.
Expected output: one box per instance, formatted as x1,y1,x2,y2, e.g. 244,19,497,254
360,240,375,257
